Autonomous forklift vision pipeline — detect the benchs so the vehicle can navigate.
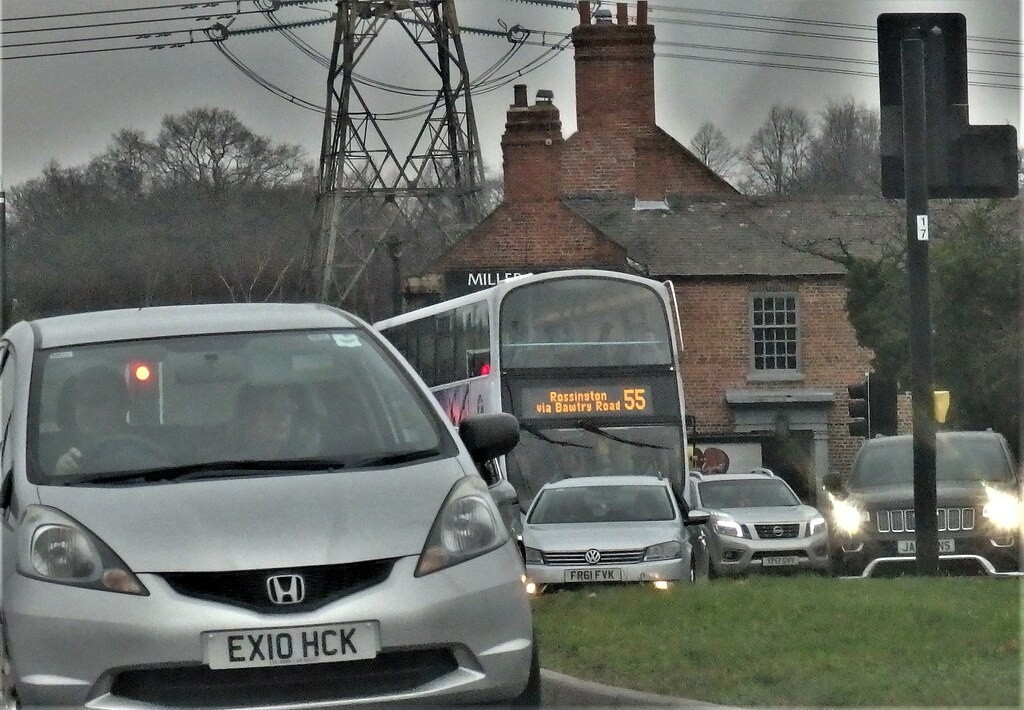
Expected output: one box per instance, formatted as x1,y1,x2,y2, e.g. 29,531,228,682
37,423,244,472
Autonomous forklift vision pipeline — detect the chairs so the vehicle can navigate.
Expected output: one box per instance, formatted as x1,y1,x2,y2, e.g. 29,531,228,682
39,379,75,477
214,384,334,463
756,488,780,507
634,489,662,519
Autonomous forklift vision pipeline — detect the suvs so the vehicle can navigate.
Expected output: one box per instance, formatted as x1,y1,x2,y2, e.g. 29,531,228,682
689,467,833,577
522,474,710,591
824,426,1023,579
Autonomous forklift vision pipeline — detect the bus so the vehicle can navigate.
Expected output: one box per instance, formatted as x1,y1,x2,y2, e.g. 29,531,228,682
374,269,690,535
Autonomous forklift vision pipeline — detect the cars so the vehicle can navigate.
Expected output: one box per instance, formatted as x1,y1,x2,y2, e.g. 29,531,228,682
0,302,546,710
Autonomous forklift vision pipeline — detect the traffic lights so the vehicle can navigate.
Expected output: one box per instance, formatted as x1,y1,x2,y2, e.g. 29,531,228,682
848,367,898,438
468,349,492,375
127,357,164,426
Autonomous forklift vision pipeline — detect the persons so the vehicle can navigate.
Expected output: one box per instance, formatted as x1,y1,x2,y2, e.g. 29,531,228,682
52,367,185,474
